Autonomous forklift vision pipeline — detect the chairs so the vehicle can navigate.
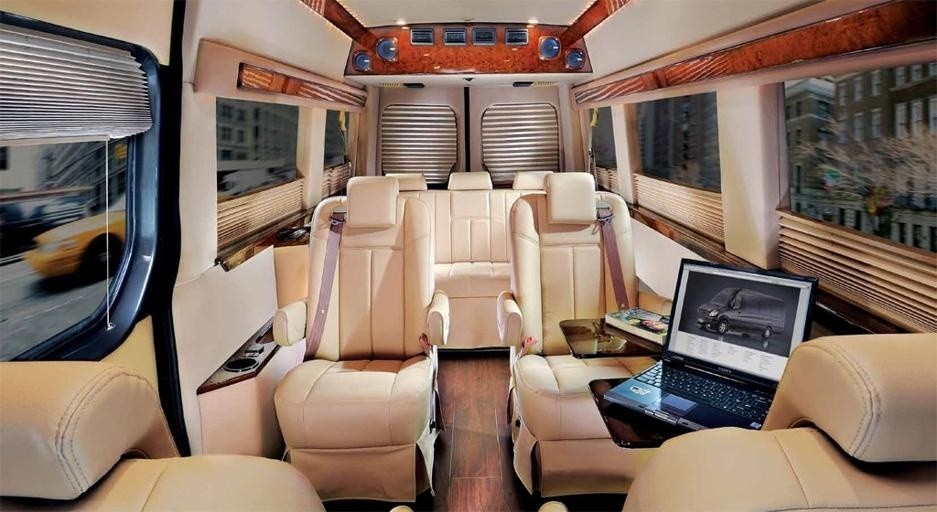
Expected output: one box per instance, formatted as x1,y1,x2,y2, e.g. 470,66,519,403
384,171,555,354
496,171,674,504
1,361,328,512
621,333,937,511
272,176,451,504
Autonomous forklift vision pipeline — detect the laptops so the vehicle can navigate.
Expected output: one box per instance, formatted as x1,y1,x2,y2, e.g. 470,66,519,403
601,256,819,445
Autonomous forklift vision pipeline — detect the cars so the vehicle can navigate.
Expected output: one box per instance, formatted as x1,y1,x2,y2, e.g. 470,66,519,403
26,184,232,277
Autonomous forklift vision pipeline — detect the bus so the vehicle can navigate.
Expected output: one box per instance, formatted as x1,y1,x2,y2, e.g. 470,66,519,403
0,185,97,266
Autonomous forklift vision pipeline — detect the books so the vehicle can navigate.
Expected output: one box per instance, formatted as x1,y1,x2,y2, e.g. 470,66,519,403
605,306,670,346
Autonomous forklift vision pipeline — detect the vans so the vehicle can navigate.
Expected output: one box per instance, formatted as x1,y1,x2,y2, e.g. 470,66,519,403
696,287,786,338
